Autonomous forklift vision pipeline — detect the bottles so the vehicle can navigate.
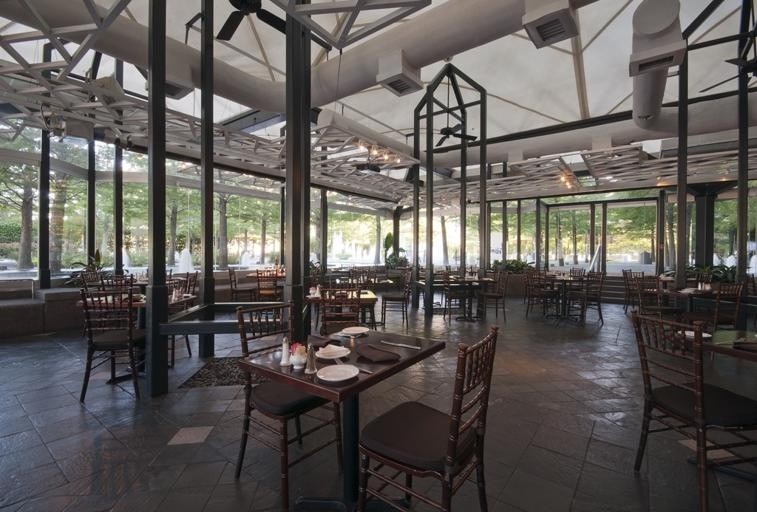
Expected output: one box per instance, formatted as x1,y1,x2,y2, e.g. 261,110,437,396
304,343,317,374
698,282,701,290
702,282,705,290
280,337,291,366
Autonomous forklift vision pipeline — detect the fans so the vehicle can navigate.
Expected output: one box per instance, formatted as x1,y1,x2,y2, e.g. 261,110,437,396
216,0,286,40
698,54,757,93
435,55,478,148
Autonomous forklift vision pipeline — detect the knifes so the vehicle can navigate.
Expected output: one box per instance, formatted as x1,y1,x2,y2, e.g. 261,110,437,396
380,340,421,351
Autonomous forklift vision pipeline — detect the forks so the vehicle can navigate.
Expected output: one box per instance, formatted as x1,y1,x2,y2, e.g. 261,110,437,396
334,359,374,374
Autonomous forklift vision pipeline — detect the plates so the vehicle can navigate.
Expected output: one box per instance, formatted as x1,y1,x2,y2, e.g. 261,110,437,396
356,291,367,294
315,326,369,382
678,291,694,294
677,330,712,340
